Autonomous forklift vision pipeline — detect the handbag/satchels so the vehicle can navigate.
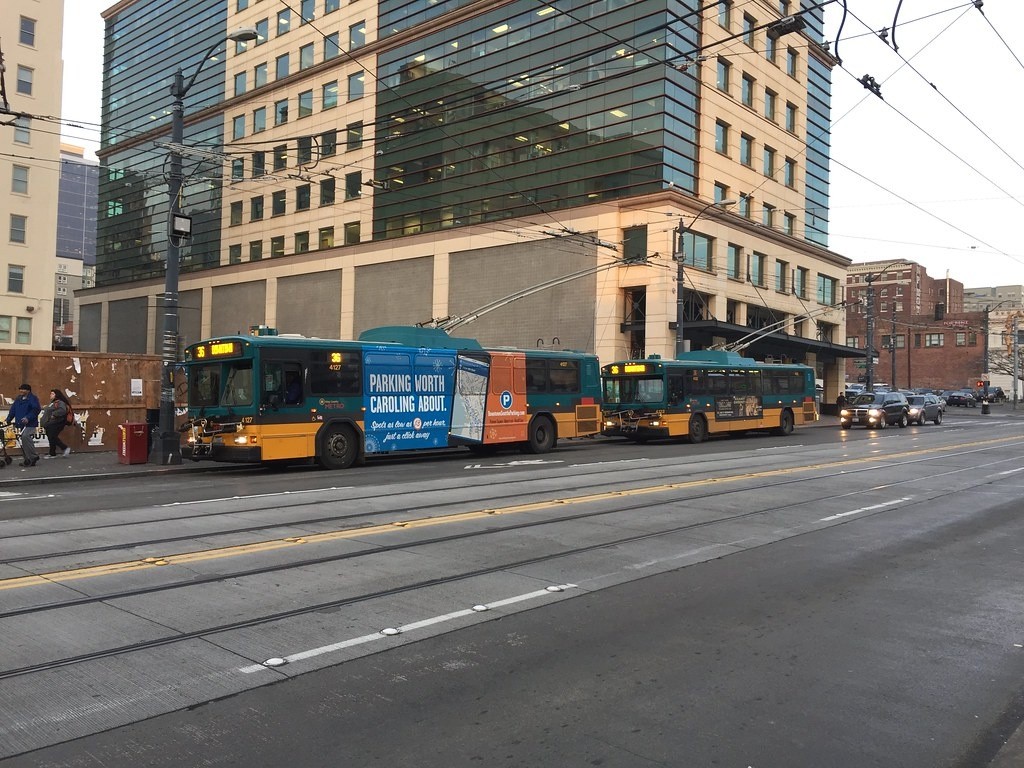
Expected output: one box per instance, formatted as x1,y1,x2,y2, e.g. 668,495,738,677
5,428,16,448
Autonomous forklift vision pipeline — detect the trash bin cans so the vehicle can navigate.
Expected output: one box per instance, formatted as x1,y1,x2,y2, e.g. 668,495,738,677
145,407,159,455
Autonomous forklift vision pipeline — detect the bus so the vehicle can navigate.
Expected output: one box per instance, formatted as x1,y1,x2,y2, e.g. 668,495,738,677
601,299,859,445
178,260,648,471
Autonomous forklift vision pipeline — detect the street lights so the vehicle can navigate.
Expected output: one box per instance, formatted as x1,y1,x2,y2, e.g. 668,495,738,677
864,259,917,389
981,299,1021,414
674,199,737,356
149,27,259,466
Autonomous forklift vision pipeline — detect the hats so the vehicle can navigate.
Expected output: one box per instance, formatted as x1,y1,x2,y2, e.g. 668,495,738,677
18,384,31,391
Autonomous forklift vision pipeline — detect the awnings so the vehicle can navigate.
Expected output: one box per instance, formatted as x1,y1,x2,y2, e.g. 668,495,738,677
670,316,878,359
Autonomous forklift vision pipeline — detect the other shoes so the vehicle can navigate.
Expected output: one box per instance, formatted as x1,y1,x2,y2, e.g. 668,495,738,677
62,447,70,457
44,455,57,459
19,460,31,466
30,456,40,466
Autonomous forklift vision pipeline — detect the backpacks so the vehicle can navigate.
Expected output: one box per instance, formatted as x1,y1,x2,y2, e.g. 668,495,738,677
55,400,73,426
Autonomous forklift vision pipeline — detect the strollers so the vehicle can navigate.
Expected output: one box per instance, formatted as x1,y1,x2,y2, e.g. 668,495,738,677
0,422,27,467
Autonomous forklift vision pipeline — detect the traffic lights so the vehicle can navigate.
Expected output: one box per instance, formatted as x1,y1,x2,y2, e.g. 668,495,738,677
977,381,985,386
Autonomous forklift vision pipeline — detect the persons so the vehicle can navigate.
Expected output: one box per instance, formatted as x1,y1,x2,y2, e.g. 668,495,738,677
836,392,848,410
264,374,299,402
3,383,41,466
41,389,71,459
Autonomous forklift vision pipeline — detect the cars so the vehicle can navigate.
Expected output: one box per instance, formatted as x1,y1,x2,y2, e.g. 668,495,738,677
816,382,1007,428
